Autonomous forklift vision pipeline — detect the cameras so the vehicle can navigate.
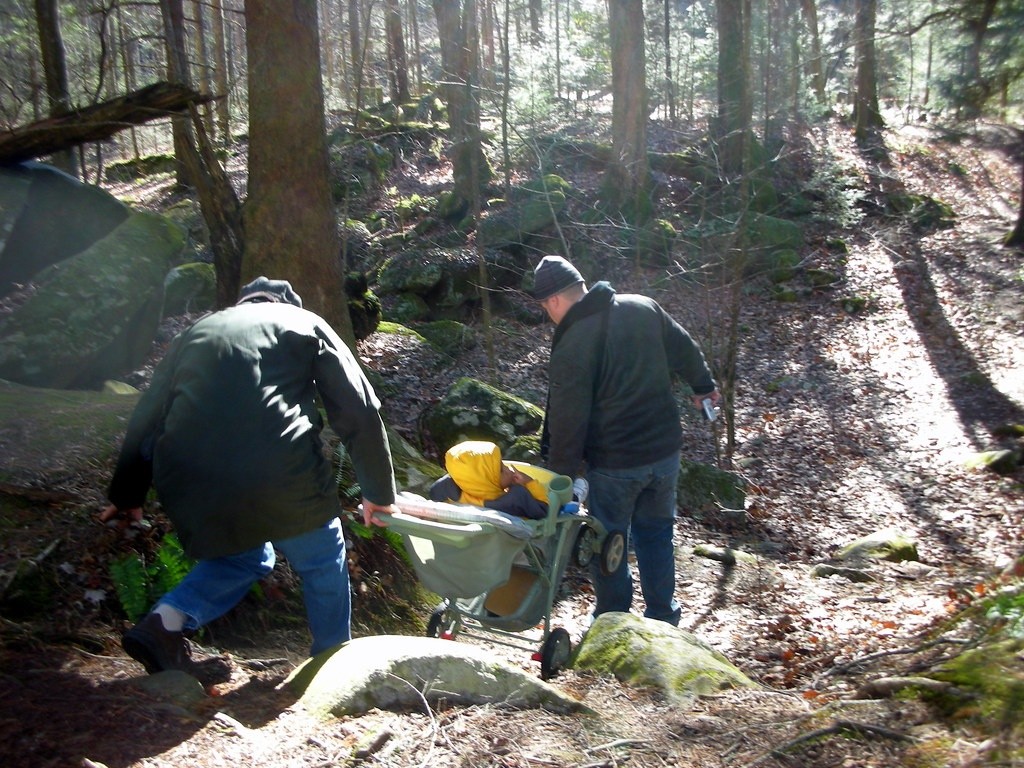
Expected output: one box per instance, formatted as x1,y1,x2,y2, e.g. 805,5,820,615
701,398,718,422
104,513,152,533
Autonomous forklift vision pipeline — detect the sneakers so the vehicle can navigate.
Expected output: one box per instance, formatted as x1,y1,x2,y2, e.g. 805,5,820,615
122,612,193,674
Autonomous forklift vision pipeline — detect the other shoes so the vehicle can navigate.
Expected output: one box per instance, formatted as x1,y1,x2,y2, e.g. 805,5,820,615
573,476,588,505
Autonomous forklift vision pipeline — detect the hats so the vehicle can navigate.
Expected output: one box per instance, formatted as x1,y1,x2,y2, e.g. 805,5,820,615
236,276,302,310
535,254,586,301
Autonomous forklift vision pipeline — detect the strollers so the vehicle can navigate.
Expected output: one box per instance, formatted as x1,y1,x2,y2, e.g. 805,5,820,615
354,454,629,683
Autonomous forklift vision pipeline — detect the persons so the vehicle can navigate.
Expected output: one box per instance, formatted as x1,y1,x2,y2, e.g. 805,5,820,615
432,441,589,632
535,255,721,624
99,276,396,675
